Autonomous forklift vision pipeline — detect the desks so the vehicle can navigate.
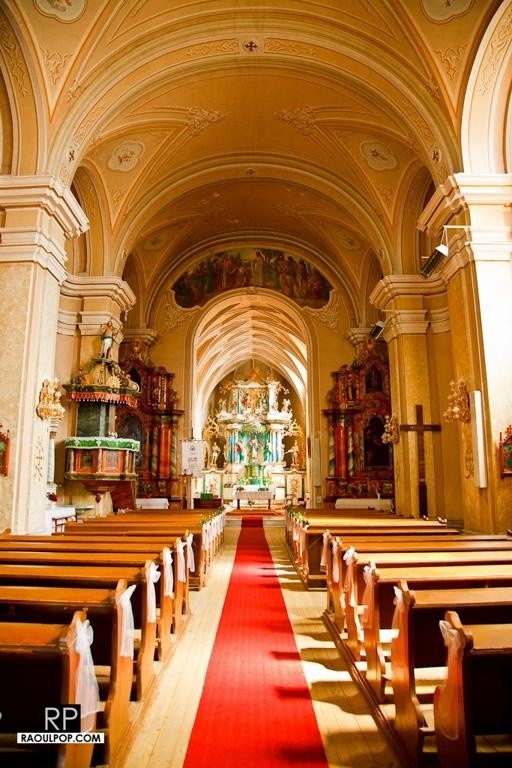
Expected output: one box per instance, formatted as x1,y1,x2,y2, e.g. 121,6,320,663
234,491,272,510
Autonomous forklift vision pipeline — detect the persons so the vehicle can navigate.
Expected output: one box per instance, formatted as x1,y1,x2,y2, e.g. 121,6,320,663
210,442,221,465
237,434,272,463
288,440,300,466
99,320,118,359
241,393,252,414
256,392,266,415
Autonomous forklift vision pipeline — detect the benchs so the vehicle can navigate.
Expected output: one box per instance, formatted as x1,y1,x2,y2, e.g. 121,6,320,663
2,505,226,767
285,505,511,766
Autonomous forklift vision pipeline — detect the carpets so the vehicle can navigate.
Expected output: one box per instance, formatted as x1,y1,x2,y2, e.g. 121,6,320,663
183,514,328,767
